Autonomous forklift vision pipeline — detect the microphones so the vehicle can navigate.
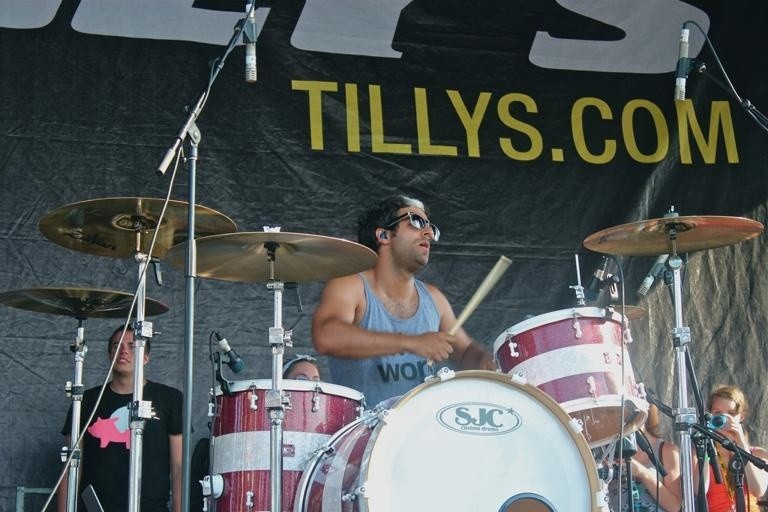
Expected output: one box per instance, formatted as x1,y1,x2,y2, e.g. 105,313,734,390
215,331,245,373
584,254,610,302
674,22,689,101
636,253,670,297
245,0,258,83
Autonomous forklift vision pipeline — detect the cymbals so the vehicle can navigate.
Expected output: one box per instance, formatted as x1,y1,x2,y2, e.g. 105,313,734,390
0,288,167,319
40,197,237,259
582,215,763,256
165,231,376,282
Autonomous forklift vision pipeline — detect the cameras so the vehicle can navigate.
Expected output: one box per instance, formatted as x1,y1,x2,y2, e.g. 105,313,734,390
709,416,726,429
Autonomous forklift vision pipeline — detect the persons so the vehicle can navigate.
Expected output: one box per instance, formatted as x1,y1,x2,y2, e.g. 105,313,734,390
609,403,682,511
311,195,494,410
690,383,768,511
55,323,183,512
282,352,322,381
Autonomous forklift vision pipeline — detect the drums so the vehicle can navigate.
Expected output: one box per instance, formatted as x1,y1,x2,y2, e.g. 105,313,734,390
495,306,648,447
294,370,603,510
203,377,364,512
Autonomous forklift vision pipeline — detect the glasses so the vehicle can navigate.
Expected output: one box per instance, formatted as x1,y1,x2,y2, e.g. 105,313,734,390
382,210,440,242
